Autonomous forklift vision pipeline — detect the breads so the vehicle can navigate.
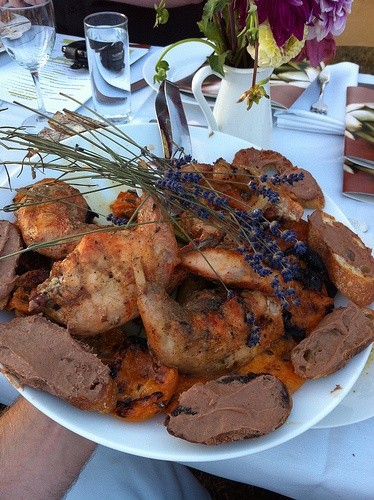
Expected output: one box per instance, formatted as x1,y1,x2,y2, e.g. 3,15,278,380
0,148,374,448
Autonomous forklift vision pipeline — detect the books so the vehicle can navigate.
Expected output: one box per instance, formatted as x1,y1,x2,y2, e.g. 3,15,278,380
174,59,374,203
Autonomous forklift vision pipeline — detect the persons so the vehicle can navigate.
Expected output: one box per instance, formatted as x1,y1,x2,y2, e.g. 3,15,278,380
1,0,206,47
1,396,213,500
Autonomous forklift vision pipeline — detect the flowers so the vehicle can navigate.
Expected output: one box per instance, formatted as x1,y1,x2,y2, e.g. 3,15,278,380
153,0,353,111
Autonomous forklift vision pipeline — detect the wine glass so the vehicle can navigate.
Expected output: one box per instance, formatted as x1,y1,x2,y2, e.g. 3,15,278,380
0,0,59,134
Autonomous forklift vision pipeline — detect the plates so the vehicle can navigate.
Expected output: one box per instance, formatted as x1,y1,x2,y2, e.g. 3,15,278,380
143,36,218,107
1,122,374,464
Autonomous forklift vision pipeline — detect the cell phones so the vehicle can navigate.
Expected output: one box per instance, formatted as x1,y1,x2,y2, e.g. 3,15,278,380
59,46,125,61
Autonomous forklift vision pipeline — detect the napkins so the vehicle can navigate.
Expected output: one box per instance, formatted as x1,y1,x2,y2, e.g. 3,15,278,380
273,62,359,135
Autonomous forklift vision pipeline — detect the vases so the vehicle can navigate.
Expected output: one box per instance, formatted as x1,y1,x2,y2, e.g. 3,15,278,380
191,65,274,150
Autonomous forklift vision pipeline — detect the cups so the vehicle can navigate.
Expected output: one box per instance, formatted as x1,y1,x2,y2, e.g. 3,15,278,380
83,12,132,124
